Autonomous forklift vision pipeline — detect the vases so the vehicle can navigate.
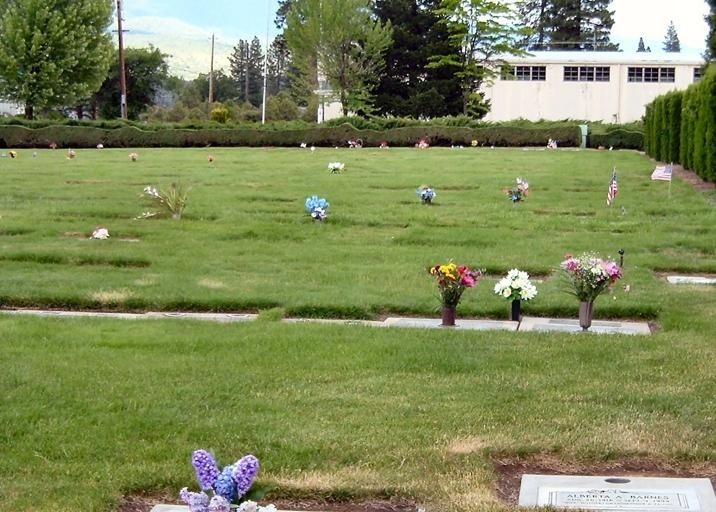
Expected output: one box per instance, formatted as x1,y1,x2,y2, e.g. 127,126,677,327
315,215,320,220
438,303,456,329
577,301,593,332
424,197,431,204
510,299,520,322
514,197,520,203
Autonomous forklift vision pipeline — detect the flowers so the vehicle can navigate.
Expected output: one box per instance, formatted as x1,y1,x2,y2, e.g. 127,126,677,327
428,259,483,308
304,195,329,220
417,183,434,203
500,178,528,202
558,251,621,300
493,266,537,302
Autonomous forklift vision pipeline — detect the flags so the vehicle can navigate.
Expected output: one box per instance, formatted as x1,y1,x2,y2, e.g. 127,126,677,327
606,174,619,205
651,164,672,182
